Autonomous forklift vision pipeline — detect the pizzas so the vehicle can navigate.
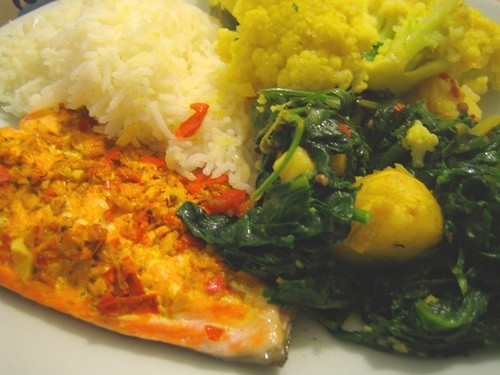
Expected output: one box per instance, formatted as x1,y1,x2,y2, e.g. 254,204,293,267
0,107,298,367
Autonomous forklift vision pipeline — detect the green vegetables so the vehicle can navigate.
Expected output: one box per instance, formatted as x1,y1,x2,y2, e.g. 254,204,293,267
176,86,500,358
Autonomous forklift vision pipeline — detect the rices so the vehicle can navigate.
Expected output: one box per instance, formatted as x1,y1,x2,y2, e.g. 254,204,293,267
0,0,260,195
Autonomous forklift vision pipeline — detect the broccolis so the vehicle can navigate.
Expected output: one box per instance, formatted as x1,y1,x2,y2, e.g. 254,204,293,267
208,0,500,124
